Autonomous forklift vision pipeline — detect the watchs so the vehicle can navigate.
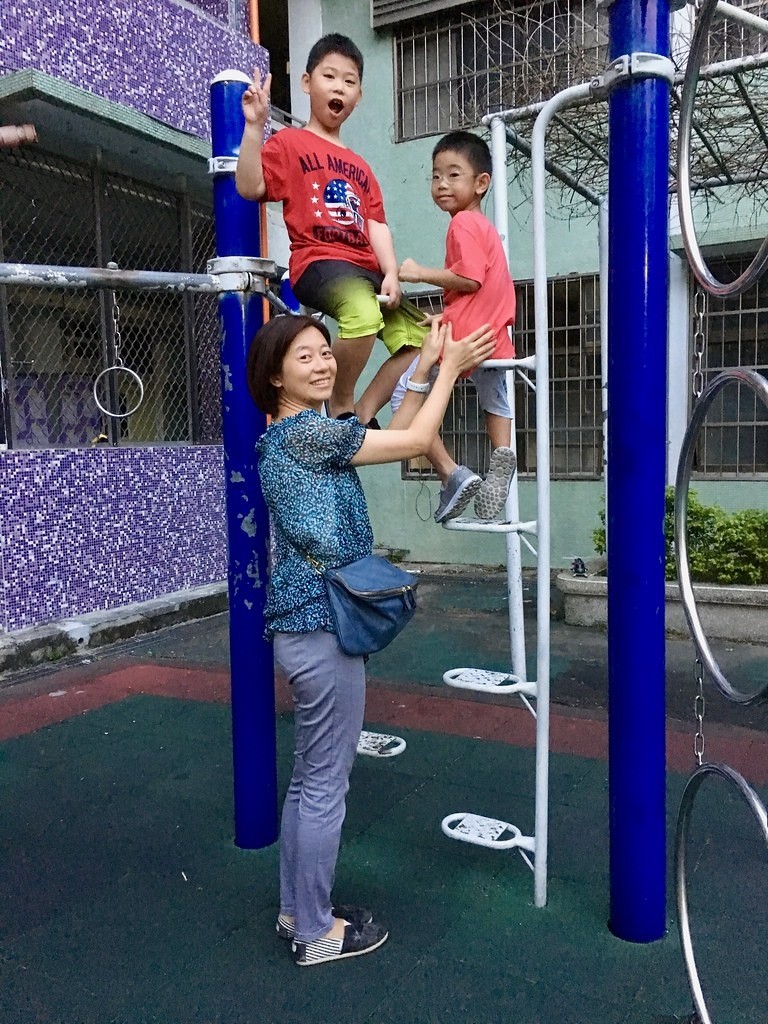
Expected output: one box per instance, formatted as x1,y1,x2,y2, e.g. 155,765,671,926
404,377,430,394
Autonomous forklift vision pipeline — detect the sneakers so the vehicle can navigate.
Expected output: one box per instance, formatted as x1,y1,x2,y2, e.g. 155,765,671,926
474,447,517,520
434,465,483,523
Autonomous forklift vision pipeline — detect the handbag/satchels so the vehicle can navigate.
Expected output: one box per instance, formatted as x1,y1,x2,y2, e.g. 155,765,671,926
324,554,419,658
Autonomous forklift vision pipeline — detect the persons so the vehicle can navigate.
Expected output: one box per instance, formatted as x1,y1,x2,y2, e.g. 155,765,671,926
246,314,498,966
234,32,432,431
398,129,518,523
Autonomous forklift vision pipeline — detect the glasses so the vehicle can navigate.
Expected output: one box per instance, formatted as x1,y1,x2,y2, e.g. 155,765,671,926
425,169,482,185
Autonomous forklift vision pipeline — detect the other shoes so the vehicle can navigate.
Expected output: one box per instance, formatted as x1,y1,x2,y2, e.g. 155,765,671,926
366,418,380,430
292,923,389,966
276,905,372,939
324,400,354,419
